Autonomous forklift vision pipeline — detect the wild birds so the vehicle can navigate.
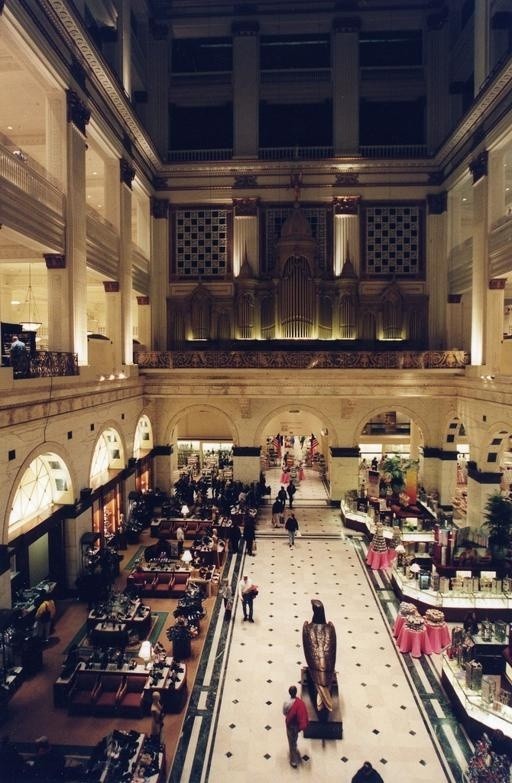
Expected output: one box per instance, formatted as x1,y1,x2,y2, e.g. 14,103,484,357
302,599,337,712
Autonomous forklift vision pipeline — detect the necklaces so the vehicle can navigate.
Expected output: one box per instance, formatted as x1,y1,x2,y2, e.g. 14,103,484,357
153,701,160,705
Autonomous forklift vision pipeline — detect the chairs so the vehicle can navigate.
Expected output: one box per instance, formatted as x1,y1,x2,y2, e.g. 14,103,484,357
133,570,191,598
159,521,212,538
66,670,150,718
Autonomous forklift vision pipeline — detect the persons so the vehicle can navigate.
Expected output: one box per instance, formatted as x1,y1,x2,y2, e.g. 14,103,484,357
222,578,234,616
151,689,166,743
284,450,290,467
286,479,296,508
283,685,310,768
278,486,288,505
243,510,256,556
9,334,28,354
285,510,299,548
238,574,258,623
351,761,383,782
271,496,284,527
33,593,56,642
